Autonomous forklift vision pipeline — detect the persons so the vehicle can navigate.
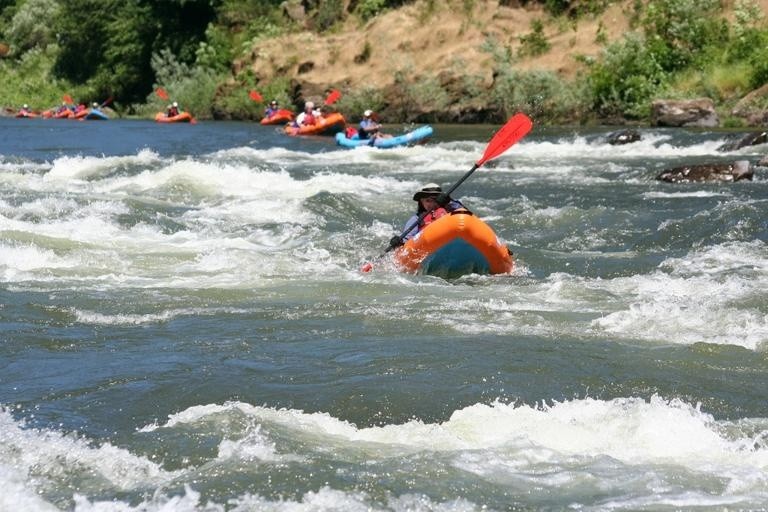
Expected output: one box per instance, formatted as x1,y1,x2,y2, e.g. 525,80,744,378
265,100,281,117
387,181,469,249
91,102,105,113
358,109,390,139
167,101,181,117
296,101,324,126
19,103,32,113
57,101,82,114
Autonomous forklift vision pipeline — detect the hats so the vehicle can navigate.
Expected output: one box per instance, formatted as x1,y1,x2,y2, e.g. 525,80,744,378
413,182,447,201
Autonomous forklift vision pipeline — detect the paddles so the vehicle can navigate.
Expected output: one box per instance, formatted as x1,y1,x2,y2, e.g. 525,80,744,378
318,90,340,110
250,92,270,108
156,88,173,106
62,95,77,109
362,113,533,271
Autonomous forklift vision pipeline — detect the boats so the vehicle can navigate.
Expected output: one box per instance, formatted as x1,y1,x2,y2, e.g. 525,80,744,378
15,111,36,118
285,112,346,136
388,206,513,283
155,111,191,123
261,109,295,126
39,107,110,120
335,125,433,149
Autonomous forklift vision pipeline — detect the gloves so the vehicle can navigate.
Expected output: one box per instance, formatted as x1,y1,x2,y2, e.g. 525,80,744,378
436,192,451,207
390,236,403,247
